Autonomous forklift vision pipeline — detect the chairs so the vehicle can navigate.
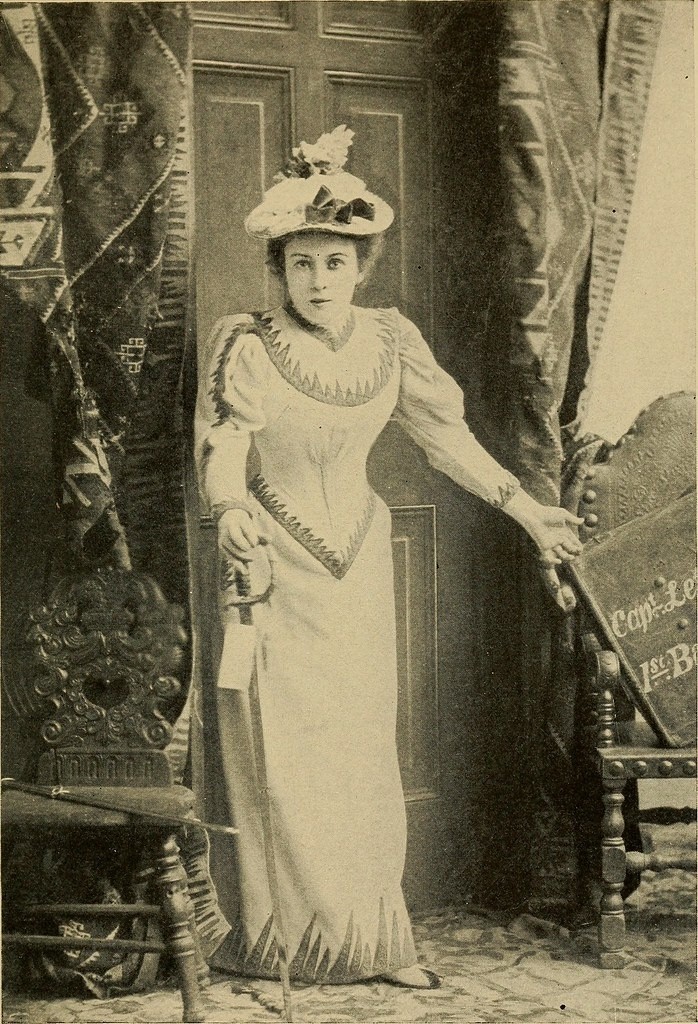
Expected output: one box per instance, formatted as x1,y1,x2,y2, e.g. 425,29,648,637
529,389,698,970
0,569,211,1024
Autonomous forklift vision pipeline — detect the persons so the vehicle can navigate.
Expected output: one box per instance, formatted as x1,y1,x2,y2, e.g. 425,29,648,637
191,124,588,991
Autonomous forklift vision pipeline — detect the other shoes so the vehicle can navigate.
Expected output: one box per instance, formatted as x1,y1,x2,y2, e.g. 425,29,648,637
380,963,444,989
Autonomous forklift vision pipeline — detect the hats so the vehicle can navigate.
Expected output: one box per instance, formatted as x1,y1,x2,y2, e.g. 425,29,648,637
245,123,394,239
